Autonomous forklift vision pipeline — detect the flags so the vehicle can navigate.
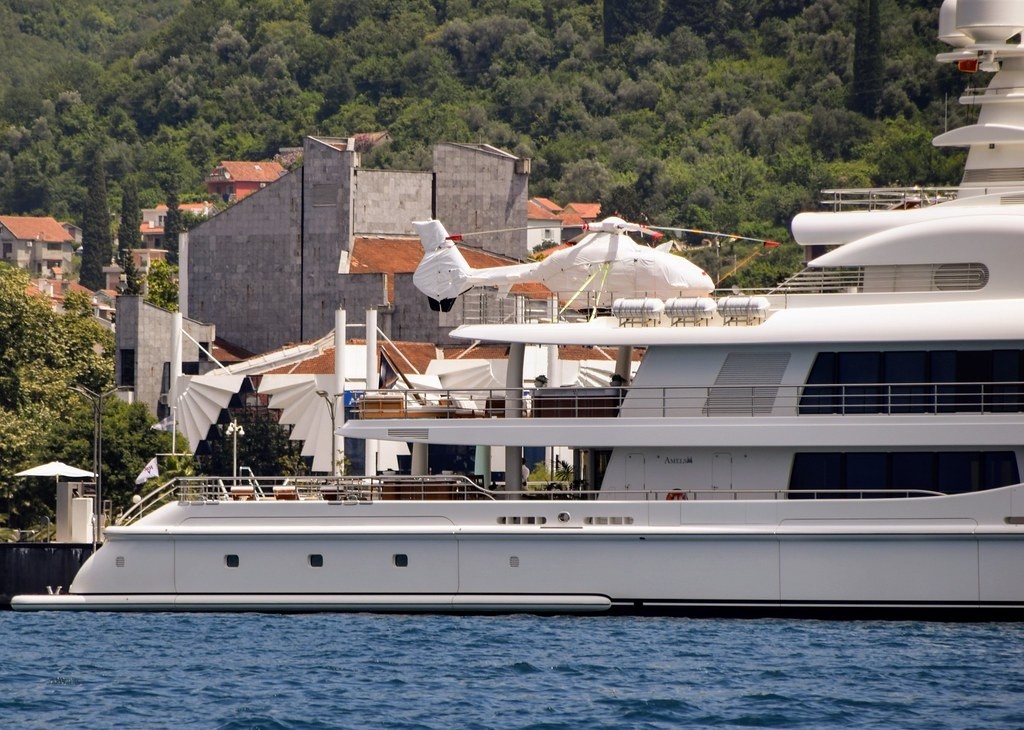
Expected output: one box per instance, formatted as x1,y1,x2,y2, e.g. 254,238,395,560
136,457,158,484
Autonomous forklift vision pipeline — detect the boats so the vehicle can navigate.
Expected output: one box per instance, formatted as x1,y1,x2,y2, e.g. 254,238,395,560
10,1,1024,623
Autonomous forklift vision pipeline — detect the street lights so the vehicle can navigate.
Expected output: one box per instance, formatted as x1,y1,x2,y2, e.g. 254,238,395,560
315,389,344,475
226,423,244,487
70,383,134,542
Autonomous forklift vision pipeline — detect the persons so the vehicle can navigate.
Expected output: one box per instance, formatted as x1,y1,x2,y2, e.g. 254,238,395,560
522,458,530,491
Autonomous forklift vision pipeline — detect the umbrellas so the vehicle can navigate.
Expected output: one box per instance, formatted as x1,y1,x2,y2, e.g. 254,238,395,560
14,461,98,483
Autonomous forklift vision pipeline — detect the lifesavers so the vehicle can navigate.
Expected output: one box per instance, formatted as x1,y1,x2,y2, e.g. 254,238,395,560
665,488,689,502
